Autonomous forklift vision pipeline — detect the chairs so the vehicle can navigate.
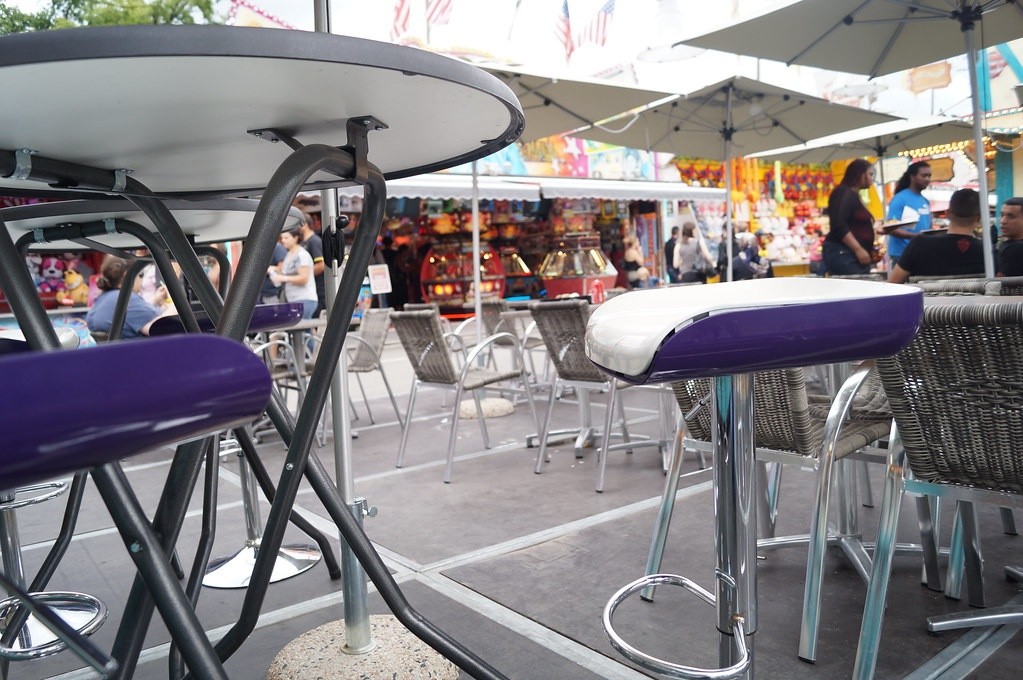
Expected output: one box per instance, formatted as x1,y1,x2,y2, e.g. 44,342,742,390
0,276,1022,680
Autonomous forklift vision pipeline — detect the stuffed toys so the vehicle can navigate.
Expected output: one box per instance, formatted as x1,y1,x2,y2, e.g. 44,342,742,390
1,196,89,306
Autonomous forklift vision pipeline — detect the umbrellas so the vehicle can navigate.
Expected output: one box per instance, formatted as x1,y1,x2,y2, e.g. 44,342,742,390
745,112,988,231
669,1,1022,279
462,65,675,141
568,76,909,282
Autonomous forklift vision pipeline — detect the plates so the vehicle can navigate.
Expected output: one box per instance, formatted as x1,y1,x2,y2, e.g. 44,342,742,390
921,229,948,235
882,220,918,229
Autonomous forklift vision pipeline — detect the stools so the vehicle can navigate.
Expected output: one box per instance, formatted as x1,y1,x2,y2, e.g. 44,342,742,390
246,301,304,440
1,332,271,678
582,276,926,679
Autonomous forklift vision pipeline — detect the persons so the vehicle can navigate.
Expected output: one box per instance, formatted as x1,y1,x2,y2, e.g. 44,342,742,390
877,161,932,282
821,159,875,275
717,220,769,282
995,197,1023,277
266,227,318,359
886,188,999,292
297,213,326,331
673,222,717,284
665,226,681,283
623,236,651,289
259,241,288,304
86,252,164,339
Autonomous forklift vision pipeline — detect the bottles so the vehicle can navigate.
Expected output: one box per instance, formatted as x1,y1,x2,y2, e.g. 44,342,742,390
267,269,282,288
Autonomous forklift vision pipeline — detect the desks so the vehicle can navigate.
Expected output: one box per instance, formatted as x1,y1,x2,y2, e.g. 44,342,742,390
0,23,526,679
249,320,322,405
